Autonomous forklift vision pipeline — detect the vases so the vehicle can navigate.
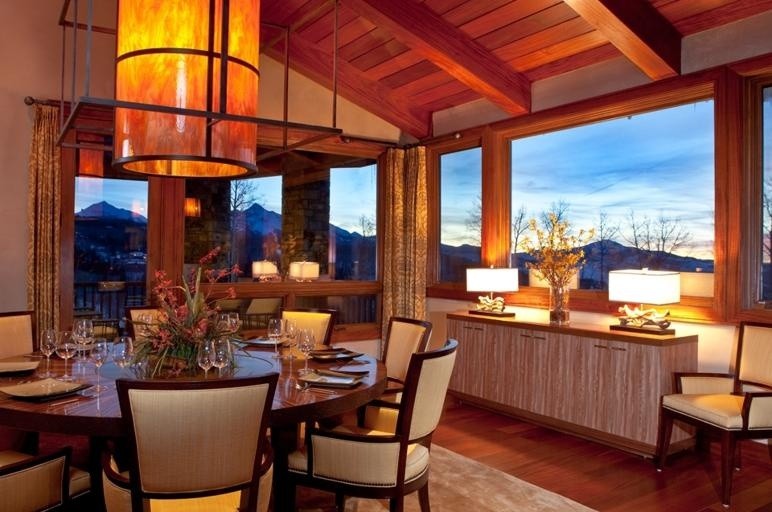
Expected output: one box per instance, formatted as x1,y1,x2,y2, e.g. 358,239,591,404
550,284,571,324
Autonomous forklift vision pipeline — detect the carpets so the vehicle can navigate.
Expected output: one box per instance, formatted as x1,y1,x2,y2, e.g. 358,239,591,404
345,443,603,512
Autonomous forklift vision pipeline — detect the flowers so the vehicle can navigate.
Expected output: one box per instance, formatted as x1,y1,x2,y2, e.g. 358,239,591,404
126,246,242,380
518,211,594,309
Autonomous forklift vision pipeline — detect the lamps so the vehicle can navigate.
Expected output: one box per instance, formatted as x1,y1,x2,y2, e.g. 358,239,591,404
184,197,201,217
608,267,680,336
679,267,715,297
466,265,519,318
252,259,279,281
528,268,580,290
288,260,319,282
54,0,342,181
75,133,105,221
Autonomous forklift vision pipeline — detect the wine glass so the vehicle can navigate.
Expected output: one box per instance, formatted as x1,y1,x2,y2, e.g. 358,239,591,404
267,318,284,357
296,328,316,374
73,318,95,362
217,313,226,321
282,319,300,359
39,329,58,377
55,331,77,379
87,337,107,392
210,339,231,377
112,336,134,378
228,313,239,321
198,342,215,376
136,313,153,333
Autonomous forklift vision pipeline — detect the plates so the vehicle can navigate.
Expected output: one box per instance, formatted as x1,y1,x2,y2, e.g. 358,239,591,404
241,333,289,348
312,349,364,361
301,369,355,390
0,376,90,398
0,360,39,373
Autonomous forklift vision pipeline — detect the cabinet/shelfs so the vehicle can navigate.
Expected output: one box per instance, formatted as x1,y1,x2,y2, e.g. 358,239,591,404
446,305,699,460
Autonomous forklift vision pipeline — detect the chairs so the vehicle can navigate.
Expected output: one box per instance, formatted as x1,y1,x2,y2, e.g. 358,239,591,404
73,307,119,342
0,310,32,357
0,423,91,512
279,306,337,346
212,298,242,312
243,298,282,329
655,321,772,508
320,317,434,428
128,307,168,340
287,339,459,512
102,372,274,512
